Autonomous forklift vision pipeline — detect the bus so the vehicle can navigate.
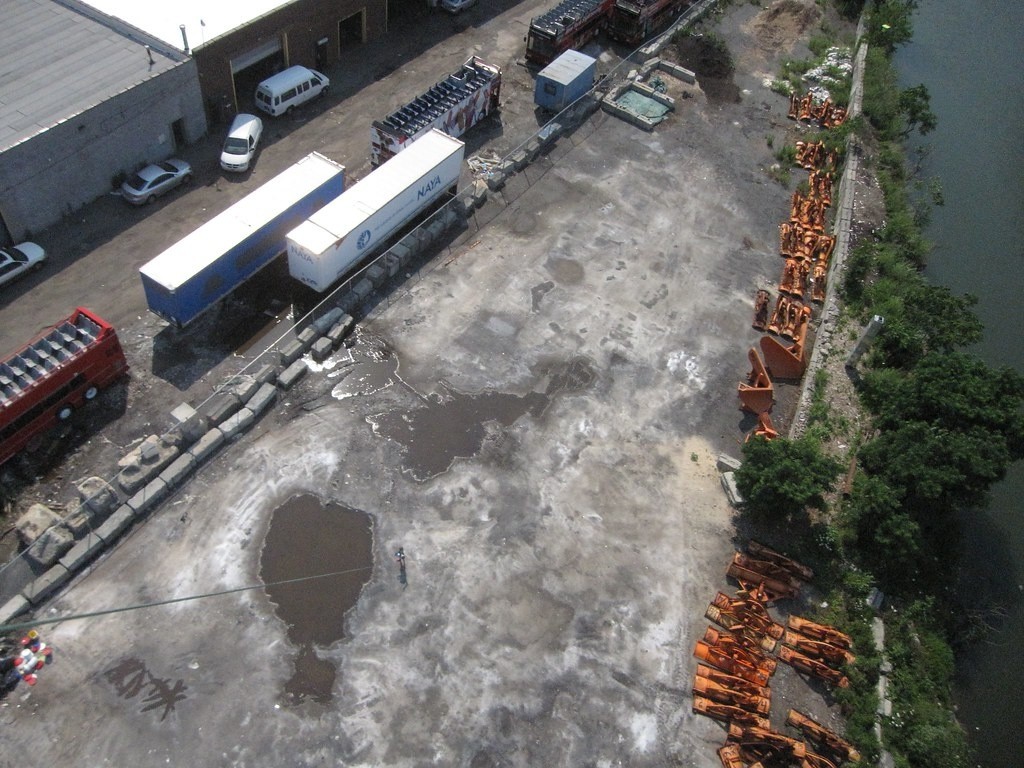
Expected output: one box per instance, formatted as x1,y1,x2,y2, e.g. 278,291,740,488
371,54,502,169
606,0,692,49
0,307,131,467
524,0,618,67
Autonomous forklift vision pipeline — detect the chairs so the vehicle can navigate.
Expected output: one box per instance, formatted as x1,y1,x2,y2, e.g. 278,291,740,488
384,60,494,136
0,313,102,409
537,0,605,32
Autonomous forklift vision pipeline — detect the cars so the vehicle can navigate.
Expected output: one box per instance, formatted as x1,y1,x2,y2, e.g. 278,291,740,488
120,158,194,206
0,242,49,292
440,0,478,16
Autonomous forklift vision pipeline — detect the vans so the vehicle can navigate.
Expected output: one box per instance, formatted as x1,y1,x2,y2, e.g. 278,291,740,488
255,65,330,116
220,114,263,173
